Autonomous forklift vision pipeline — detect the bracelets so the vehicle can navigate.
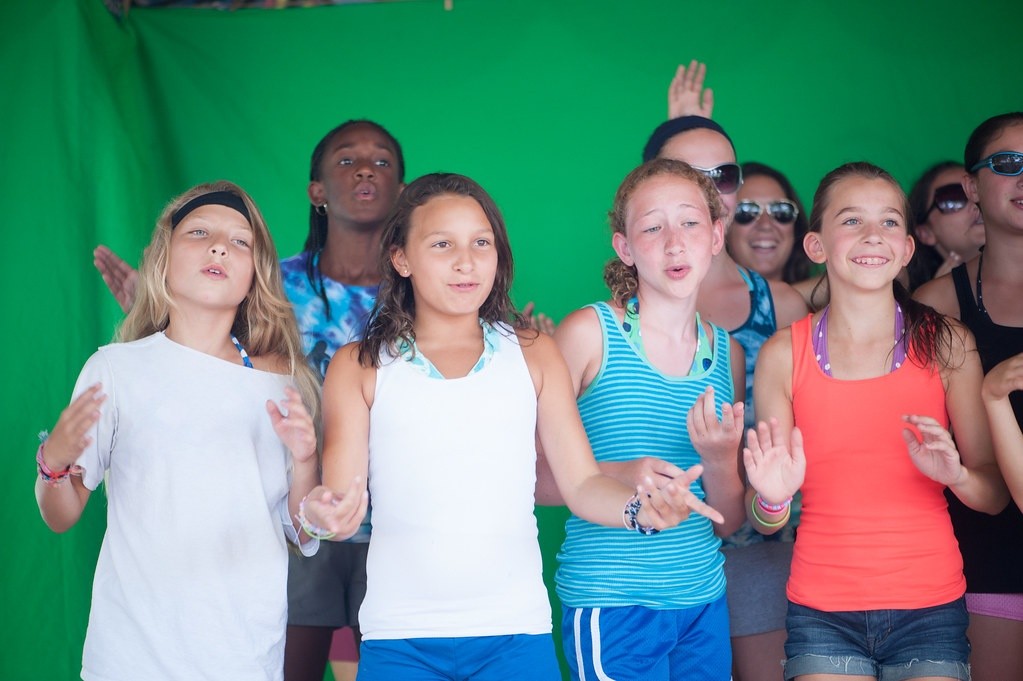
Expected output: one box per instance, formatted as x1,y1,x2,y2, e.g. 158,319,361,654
756,495,793,516
624,494,660,536
296,521,333,541
622,492,635,532
751,493,790,528
35,430,87,486
299,496,335,536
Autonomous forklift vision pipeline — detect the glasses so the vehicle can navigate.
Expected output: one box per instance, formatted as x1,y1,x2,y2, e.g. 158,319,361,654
734,199,799,225
692,163,742,194
921,184,970,222
968,151,1023,175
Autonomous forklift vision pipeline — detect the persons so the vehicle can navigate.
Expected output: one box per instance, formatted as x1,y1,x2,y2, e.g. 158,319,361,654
32,58,1023,681
